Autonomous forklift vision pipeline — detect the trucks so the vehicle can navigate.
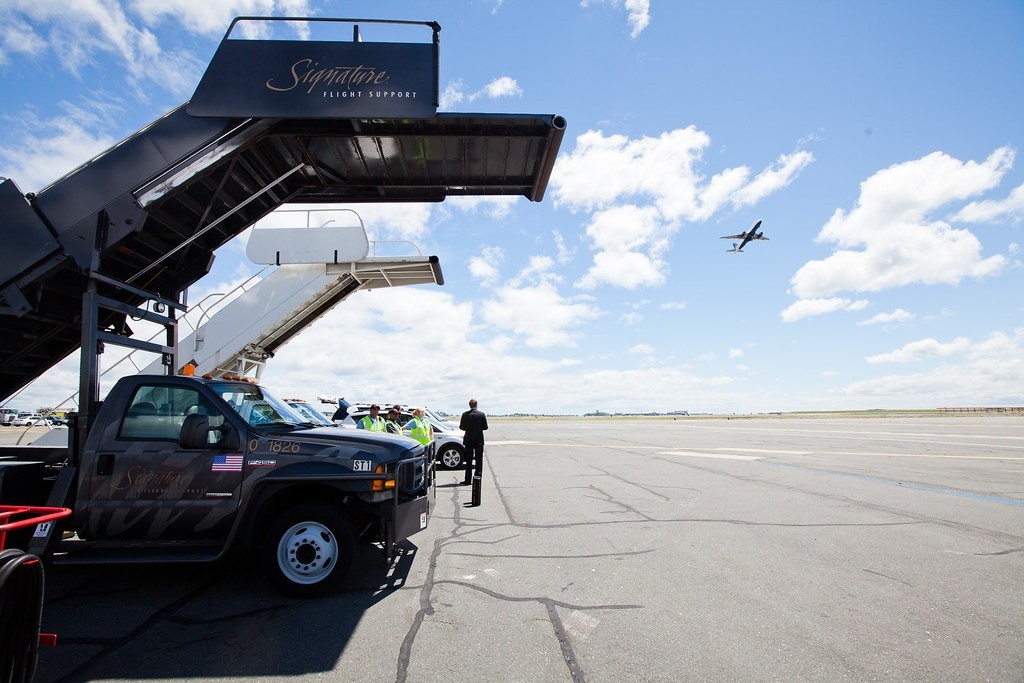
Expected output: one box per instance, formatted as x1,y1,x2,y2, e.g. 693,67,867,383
0,408,15,425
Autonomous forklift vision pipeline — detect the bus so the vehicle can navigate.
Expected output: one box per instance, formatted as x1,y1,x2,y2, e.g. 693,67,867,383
49,412,67,418
17,412,43,418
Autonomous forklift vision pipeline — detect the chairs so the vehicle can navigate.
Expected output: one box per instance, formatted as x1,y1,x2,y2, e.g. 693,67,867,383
116,403,218,443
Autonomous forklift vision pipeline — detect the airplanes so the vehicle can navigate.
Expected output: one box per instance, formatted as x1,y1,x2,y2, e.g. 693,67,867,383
717,220,770,253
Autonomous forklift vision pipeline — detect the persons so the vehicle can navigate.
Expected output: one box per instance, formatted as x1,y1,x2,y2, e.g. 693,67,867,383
385,405,403,436
459,399,488,485
353,404,386,432
402,408,433,447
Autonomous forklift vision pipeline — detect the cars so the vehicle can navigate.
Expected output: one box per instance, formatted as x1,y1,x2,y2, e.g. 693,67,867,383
47,416,68,426
313,400,476,470
13,416,53,426
239,399,336,427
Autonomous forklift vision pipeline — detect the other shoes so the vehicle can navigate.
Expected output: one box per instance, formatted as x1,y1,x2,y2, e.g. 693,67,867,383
460,480,471,485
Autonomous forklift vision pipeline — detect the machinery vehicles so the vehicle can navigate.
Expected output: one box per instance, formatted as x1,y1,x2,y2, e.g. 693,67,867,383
0,14,566,602
12,208,446,449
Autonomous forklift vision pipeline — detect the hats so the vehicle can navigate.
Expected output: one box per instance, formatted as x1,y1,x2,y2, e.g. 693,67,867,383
370,404,380,410
388,409,401,414
